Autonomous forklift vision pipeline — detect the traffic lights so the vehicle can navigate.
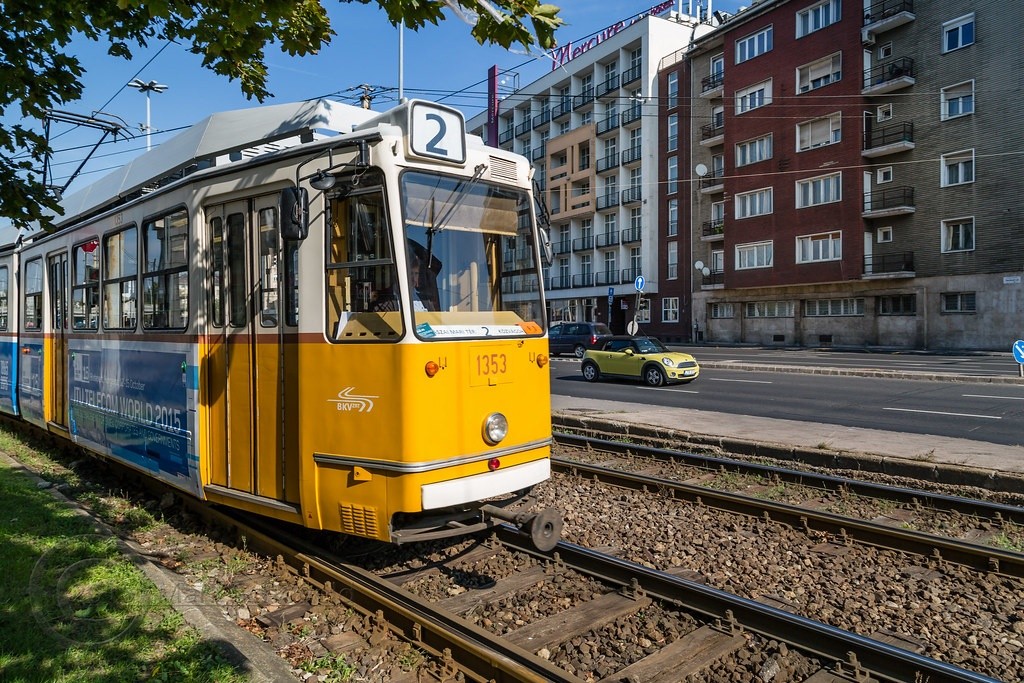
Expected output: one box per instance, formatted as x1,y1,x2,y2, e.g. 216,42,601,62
86,264,99,308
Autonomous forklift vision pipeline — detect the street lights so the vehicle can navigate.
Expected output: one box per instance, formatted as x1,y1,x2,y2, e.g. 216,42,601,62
127,78,168,152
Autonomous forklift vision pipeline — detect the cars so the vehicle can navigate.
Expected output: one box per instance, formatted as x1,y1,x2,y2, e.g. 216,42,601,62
581,335,700,387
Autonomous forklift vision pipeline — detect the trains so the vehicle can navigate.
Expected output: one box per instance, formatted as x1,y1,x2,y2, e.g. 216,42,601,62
0,107,563,552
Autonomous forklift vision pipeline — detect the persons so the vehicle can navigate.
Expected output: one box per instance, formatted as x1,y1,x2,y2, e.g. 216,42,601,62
372,257,436,312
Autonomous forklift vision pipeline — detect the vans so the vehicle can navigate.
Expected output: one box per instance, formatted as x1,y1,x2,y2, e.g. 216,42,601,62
549,322,613,358
